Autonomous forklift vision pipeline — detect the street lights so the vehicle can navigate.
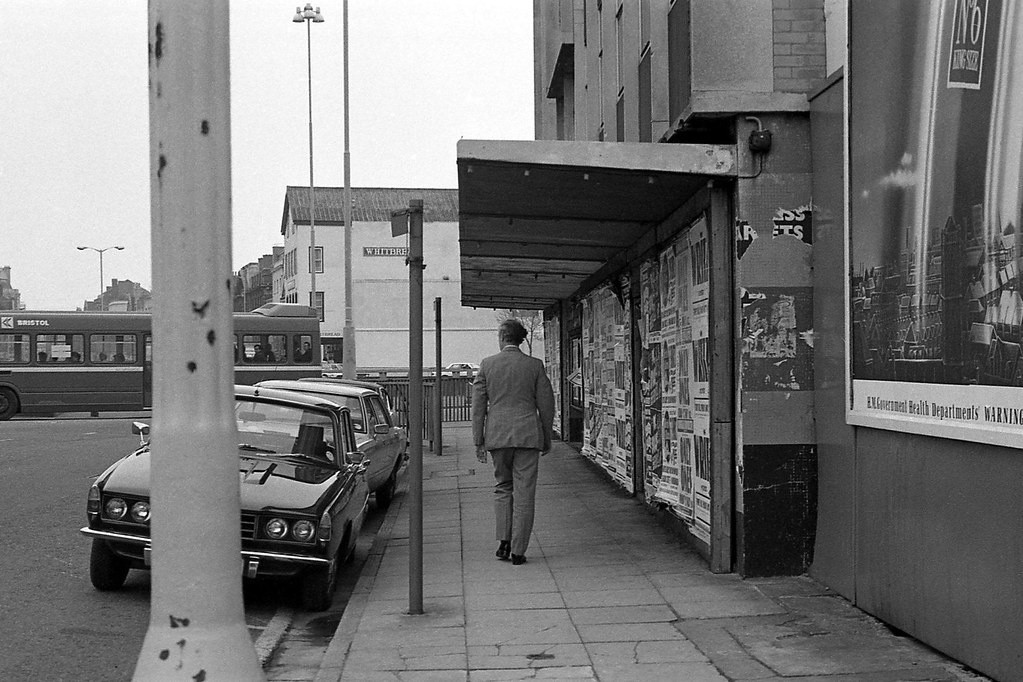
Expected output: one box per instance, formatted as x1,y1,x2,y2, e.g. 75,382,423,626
77,245,129,352
290,3,326,315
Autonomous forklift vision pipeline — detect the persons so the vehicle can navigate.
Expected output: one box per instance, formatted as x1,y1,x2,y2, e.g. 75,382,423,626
294,341,312,363
243,343,252,363
99,351,108,362
323,345,336,363
64,351,81,363
112,353,125,363
252,344,265,363
471,320,555,562
265,344,276,362
38,351,48,362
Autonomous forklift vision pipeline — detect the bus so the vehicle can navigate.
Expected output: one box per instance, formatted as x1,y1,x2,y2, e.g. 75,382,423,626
318,337,345,379
0,302,322,423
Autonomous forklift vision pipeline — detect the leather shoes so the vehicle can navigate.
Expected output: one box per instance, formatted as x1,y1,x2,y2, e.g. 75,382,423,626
511,552,526,565
497,542,511,559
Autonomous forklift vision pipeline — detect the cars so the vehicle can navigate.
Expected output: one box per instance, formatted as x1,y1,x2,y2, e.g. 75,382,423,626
296,377,394,432
80,385,372,612
229,380,411,510
